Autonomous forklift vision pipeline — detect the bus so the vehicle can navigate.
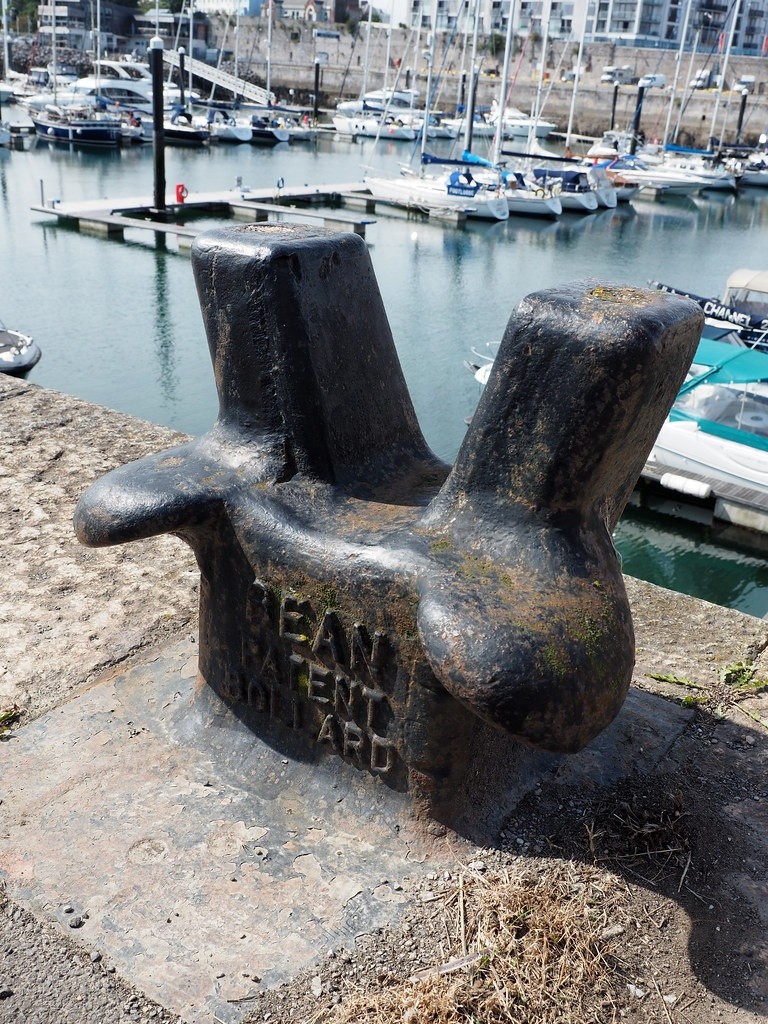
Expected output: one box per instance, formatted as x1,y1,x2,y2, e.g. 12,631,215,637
313,29,340,39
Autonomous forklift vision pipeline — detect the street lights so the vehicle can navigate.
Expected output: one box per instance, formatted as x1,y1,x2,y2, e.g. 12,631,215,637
462,70,467,105
611,81,620,130
468,66,479,152
406,67,410,89
150,37,166,209
630,78,646,155
736,89,748,144
314,58,320,121
178,47,186,105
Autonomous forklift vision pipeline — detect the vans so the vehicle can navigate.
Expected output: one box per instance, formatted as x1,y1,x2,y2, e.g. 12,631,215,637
641,74,665,89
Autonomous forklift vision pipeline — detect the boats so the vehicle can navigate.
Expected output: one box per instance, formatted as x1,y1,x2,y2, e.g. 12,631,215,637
0,327,41,378
463,269,768,552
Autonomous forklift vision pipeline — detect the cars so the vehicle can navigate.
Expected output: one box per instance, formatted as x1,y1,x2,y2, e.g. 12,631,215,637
14,37,42,46
561,73,580,83
734,81,755,94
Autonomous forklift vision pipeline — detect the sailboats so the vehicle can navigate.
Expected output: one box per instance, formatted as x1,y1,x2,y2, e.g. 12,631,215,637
363,1,768,220
0,0,559,147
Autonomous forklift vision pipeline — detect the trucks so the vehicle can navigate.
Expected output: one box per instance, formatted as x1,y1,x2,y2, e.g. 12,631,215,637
601,67,632,84
689,70,713,90
